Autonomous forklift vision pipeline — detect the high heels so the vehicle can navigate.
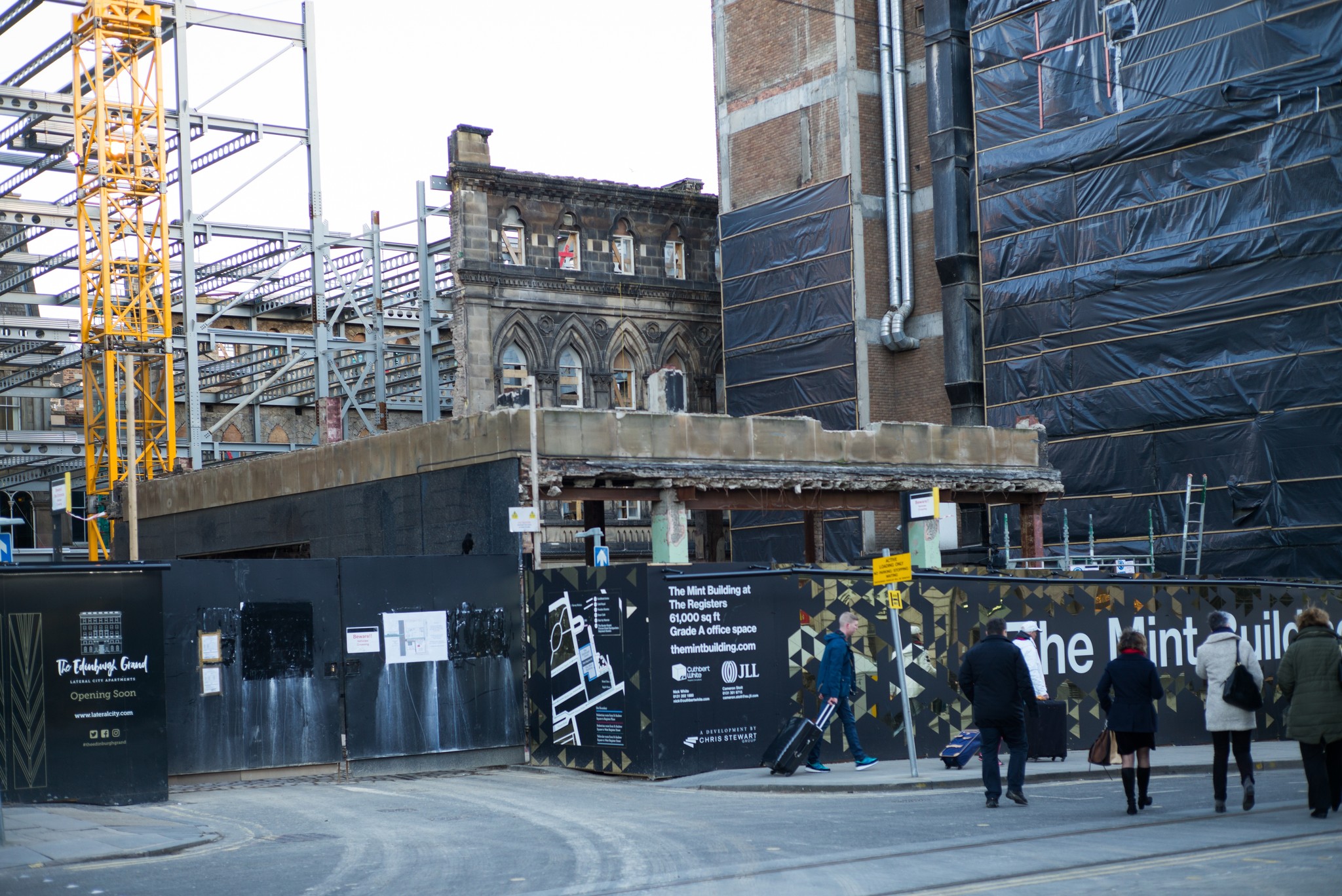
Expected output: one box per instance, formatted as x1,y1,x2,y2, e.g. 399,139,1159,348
1243,778,1255,811
1215,799,1227,813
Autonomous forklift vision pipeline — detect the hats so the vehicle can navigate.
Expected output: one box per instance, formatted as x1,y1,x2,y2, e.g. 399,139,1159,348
1022,620,1043,633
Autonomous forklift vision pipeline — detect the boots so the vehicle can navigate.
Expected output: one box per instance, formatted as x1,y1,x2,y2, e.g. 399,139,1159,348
1137,765,1153,810
1121,767,1138,816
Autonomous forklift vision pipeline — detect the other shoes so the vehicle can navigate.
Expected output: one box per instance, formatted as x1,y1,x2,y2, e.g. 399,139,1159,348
1311,804,1328,818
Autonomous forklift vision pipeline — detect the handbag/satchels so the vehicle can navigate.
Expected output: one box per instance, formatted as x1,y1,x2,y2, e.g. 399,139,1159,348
1088,720,1122,766
1219,664,1264,712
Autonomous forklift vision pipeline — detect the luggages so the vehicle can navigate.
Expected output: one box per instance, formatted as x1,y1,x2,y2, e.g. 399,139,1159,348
939,729,983,770
759,700,838,777
1024,695,1068,762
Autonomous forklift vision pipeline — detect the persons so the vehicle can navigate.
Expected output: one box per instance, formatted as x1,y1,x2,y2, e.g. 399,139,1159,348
804,611,879,772
1196,610,1263,812
1277,608,1342,818
958,618,1040,808
979,621,1049,766
1096,629,1165,815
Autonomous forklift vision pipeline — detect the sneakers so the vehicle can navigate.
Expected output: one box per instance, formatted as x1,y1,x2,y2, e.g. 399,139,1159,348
804,760,830,773
985,798,999,807
978,752,983,761
998,758,1005,766
854,756,879,771
1005,789,1028,805
